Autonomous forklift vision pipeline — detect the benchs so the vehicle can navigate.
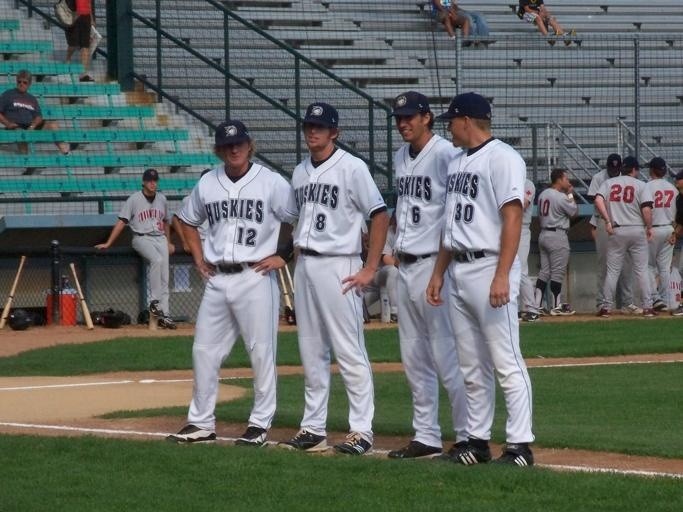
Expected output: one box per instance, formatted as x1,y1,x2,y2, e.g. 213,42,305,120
0,0,683,216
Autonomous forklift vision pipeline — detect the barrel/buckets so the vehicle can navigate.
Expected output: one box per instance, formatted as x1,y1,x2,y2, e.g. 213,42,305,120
380,286,391,323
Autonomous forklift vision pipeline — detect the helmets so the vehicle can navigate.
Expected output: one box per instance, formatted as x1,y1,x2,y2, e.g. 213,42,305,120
8,310,31,330
101,311,123,329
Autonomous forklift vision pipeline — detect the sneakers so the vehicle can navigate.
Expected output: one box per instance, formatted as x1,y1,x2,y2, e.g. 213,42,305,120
563,26,576,46
333,432,374,456
149,303,164,317
522,304,576,322
549,30,558,46
235,426,269,448
597,300,683,318
387,440,533,468
165,425,216,444
277,429,329,453
160,318,176,329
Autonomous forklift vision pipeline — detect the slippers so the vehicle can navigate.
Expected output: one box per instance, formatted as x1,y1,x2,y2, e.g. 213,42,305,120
80,74,94,81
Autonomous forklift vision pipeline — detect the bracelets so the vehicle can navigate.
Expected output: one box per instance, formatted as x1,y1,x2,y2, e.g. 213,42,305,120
568,193,573,199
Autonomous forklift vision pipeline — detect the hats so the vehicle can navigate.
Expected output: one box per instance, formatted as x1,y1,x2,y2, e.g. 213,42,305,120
215,120,250,146
302,103,339,129
436,92,492,120
143,169,159,181
607,154,683,178
386,91,430,118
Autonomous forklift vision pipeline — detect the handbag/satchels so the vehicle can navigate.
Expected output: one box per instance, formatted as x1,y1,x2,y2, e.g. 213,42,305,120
54,0,79,28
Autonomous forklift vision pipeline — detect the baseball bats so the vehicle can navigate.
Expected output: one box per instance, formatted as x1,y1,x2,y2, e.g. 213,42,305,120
70,262,95,329
0,255,26,330
279,264,295,323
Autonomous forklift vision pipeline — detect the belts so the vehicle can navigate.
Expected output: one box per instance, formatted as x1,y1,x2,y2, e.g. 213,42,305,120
545,228,556,232
455,251,484,262
397,253,430,263
217,262,256,273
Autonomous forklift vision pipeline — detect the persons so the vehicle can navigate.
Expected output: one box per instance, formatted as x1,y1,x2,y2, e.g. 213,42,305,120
93,168,178,329
386,92,469,460
371,209,398,324
641,156,679,311
587,153,643,315
63,0,96,81
431,1,469,40
518,0,571,46
537,168,576,317
172,168,209,253
594,156,658,317
280,102,389,456
426,93,535,466
517,179,539,320
167,120,299,449
668,170,683,245
1,69,71,154
